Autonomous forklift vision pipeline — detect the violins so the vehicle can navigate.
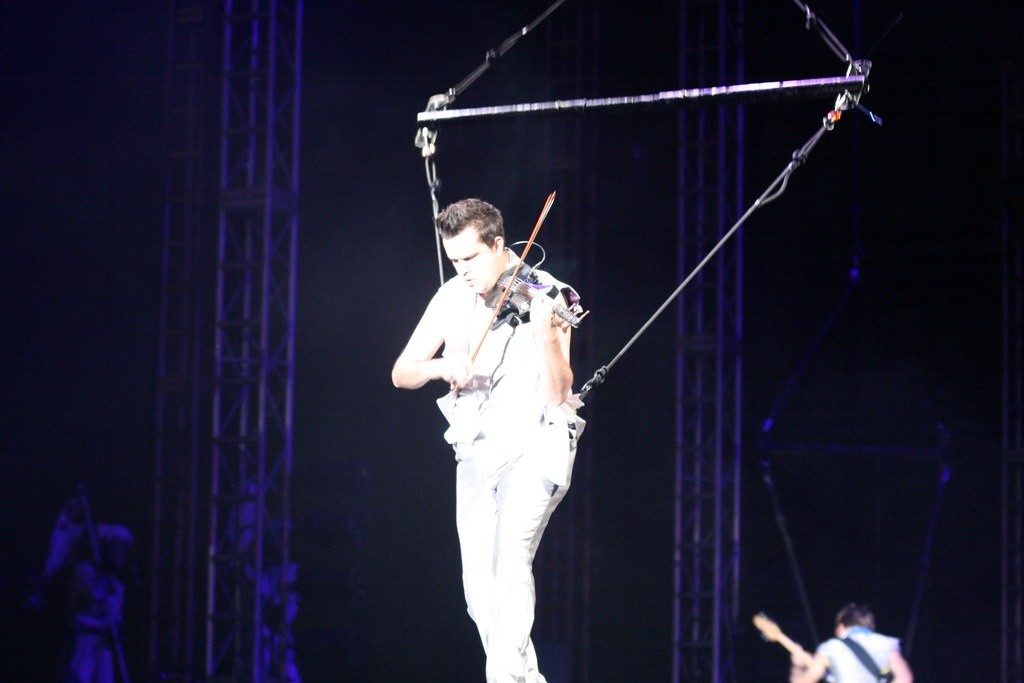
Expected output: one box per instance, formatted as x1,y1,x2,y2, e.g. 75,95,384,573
486,265,590,335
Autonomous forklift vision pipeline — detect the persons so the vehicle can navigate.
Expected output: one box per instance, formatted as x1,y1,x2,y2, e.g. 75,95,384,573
391,196,585,683
215,501,302,683
62,522,134,683
790,605,914,683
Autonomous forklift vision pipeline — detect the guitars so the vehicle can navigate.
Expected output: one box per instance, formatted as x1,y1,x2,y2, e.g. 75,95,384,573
751,613,835,683
